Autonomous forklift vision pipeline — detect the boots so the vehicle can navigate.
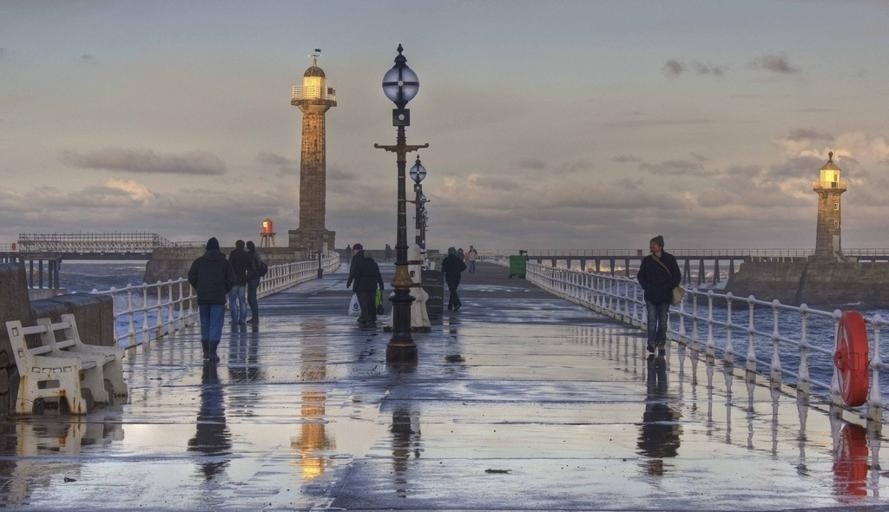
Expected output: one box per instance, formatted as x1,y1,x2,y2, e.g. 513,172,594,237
202,340,220,362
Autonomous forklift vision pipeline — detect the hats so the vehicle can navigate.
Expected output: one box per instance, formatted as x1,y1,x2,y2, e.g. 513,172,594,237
650,235,664,247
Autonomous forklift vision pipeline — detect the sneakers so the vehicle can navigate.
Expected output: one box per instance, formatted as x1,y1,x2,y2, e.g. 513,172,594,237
647,355,665,363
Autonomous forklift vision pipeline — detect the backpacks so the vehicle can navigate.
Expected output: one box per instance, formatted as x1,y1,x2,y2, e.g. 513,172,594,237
258,260,268,276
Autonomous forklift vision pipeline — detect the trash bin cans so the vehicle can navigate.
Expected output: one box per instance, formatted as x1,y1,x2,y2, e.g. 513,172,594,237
421,270,443,316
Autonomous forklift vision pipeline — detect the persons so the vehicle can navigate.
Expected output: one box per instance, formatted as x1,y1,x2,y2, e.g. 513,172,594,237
228,240,262,326
457,245,478,273
187,361,231,480
346,243,352,264
346,244,384,326
637,355,681,478
188,237,234,362
637,235,682,356
442,245,467,312
384,244,392,263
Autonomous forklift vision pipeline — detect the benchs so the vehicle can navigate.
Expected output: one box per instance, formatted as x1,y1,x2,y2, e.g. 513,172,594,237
5,313,127,415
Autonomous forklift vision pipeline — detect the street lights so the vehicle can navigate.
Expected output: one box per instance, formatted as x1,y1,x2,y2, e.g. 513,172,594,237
373,40,430,372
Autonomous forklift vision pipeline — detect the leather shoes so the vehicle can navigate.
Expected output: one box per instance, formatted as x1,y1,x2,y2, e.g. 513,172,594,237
647,340,666,354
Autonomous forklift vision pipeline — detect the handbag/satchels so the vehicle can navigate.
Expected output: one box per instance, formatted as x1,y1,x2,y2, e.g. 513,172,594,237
671,286,685,305
376,289,384,315
348,293,362,317
